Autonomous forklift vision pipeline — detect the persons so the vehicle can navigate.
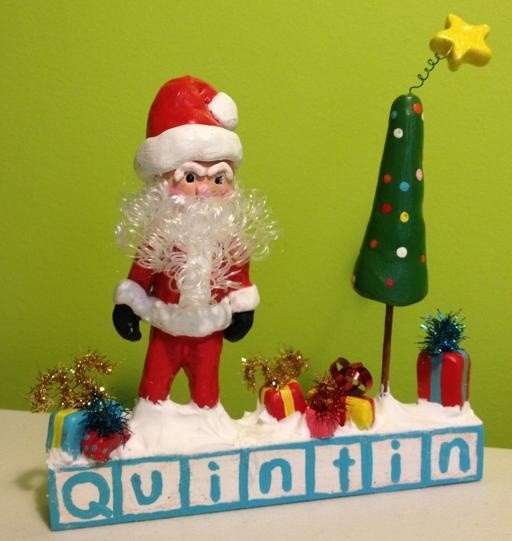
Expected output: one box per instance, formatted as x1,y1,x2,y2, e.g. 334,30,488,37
111,70,259,409
